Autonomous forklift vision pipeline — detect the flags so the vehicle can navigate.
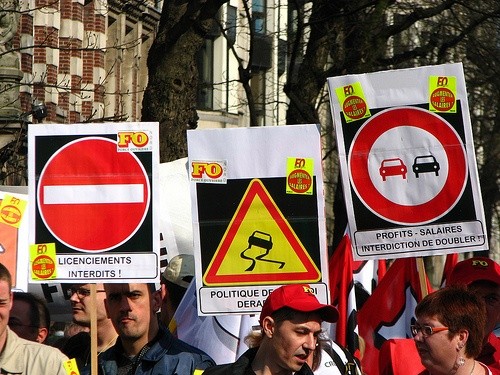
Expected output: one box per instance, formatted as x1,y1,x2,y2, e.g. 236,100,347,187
357,257,434,375
326,213,388,349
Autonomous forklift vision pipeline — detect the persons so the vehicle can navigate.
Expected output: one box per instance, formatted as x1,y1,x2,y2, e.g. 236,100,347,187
445,257,500,370
0,255,339,375
409,286,500,375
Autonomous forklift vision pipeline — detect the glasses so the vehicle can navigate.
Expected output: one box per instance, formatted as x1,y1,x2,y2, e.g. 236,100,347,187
67,289,106,298
8,317,40,330
410,325,450,338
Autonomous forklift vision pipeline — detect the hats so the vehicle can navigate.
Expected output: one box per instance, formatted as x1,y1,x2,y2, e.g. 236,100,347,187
162,254,195,288
260,283,339,328
447,257,500,290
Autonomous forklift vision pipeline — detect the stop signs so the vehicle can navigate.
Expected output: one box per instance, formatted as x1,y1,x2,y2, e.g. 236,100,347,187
37,136,150,254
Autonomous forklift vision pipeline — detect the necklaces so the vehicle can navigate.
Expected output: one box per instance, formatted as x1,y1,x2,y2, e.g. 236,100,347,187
469,359,477,375
